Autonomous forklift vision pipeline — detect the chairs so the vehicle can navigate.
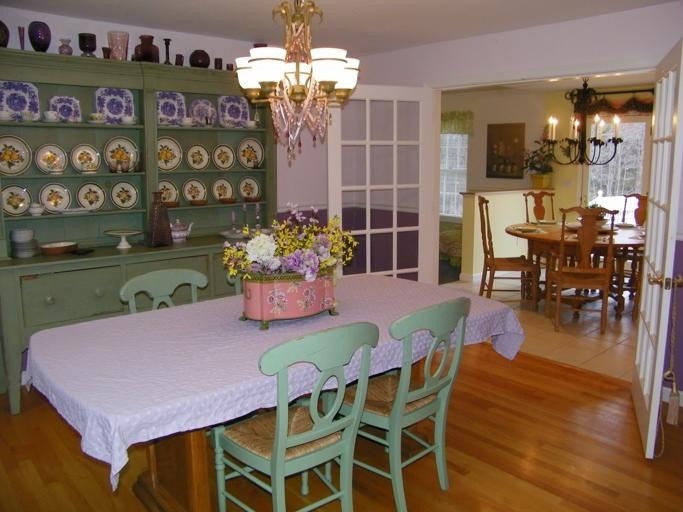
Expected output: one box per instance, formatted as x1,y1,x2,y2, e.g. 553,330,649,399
317,291,472,512
202,321,381,512
120,267,209,315
474,189,653,334
224,259,257,295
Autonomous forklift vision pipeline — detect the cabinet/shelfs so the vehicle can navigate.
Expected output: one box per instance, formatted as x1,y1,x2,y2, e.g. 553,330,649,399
0,234,236,417
0,53,276,264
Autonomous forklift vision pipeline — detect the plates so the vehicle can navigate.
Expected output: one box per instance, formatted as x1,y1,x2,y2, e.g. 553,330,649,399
74,180,107,212
157,136,183,172
1,134,34,178
37,182,72,214
103,136,140,175
108,180,140,211
615,223,637,228
180,177,207,206
184,143,210,171
54,205,96,215
47,95,83,123
1,184,33,216
70,142,102,175
156,91,187,127
210,143,235,171
235,136,265,169
515,226,538,233
158,179,179,208
41,239,78,258
210,176,234,204
219,228,272,239
218,95,251,129
1,82,41,125
237,176,261,202
94,86,136,126
34,141,70,176
188,98,217,128
539,220,558,225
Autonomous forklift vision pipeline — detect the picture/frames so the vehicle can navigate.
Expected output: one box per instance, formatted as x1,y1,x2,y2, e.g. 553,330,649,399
484,118,527,181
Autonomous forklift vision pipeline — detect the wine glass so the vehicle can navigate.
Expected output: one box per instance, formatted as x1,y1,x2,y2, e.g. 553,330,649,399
78,33,98,58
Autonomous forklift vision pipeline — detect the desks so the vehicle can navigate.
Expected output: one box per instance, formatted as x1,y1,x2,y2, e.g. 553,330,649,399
21,272,526,512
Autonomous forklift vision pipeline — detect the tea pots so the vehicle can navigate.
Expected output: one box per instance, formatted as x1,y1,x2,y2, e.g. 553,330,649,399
169,218,195,244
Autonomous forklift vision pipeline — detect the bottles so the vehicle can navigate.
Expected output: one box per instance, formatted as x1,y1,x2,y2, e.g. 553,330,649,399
148,190,170,248
134,34,162,63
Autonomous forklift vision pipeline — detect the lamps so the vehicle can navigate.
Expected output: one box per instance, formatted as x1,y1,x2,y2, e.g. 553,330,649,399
233,1,367,154
542,78,623,167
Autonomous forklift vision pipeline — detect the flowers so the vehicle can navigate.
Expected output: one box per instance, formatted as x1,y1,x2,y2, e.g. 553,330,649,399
220,197,360,283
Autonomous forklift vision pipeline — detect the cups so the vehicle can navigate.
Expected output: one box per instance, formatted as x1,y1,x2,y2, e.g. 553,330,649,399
106,30,130,62
87,111,107,124
180,117,196,127
244,119,260,129
43,110,61,123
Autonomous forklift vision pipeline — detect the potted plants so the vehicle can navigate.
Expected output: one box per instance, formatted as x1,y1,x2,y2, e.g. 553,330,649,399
522,130,557,189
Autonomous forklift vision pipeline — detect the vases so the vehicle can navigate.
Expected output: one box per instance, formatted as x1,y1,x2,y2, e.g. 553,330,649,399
0,19,224,71
238,272,341,332
145,190,175,249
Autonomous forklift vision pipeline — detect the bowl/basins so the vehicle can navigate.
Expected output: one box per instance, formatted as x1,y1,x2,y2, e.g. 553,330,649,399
48,167,66,177
26,200,46,218
218,196,237,205
188,199,208,206
159,200,180,208
243,196,261,204
9,227,41,262
79,167,100,175
